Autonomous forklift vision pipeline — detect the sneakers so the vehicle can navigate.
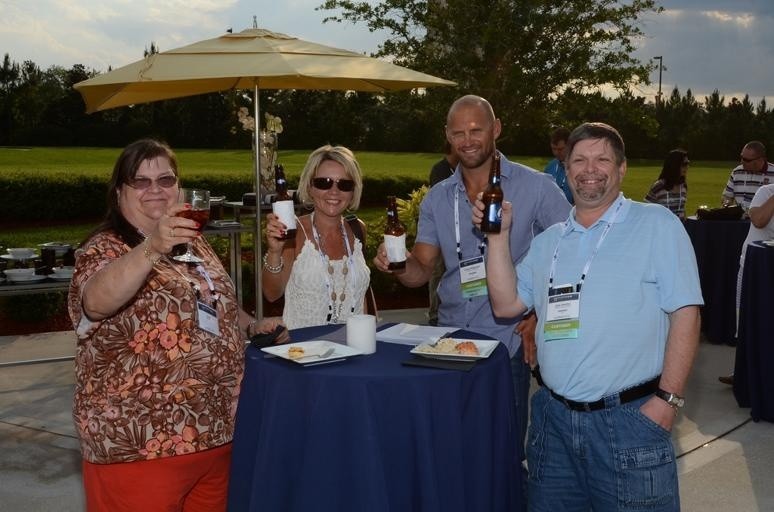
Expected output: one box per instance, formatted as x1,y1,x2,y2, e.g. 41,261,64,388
718,373,734,385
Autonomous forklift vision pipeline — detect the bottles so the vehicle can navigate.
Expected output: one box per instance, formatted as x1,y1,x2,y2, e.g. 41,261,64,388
272,164,299,240
385,195,407,272
483,150,506,237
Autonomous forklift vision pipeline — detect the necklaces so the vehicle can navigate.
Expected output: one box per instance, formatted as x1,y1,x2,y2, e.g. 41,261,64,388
313,220,348,324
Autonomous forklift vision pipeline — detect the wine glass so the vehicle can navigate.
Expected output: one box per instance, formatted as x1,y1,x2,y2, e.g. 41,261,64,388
170,185,210,264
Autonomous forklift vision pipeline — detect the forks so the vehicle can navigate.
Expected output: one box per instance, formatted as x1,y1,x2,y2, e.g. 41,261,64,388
288,347,335,361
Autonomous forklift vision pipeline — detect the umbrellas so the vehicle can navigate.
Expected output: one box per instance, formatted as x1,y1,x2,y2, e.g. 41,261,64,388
73,16,457,315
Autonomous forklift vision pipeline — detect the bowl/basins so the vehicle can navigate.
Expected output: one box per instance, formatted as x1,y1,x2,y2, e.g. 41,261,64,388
51,265,75,277
41,242,70,256
2,269,35,279
6,247,38,257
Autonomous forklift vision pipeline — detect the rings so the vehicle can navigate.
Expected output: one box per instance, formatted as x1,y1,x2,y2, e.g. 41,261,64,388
170,230,174,237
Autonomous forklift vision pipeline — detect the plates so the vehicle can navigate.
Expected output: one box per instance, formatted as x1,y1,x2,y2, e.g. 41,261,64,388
409,335,502,365
261,339,360,368
8,276,48,283
48,273,73,283
0,278,6,285
0,254,40,260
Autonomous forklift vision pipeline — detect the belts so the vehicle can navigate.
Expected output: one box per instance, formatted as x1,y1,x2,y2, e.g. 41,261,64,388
533,362,666,413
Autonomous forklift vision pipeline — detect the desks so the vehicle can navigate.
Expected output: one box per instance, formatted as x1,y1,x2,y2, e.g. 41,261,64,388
684,211,753,346
725,239,774,426
199,218,257,307
224,318,523,512
219,196,323,320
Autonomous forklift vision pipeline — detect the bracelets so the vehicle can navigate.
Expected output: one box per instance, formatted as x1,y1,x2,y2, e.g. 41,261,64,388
142,236,164,266
246,320,255,340
263,253,285,275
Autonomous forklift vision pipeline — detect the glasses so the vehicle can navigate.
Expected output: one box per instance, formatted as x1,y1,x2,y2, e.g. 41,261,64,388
739,155,762,163
682,159,691,168
309,177,357,193
125,174,177,190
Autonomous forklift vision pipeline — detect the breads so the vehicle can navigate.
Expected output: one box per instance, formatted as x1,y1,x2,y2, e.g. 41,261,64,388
456,342,479,355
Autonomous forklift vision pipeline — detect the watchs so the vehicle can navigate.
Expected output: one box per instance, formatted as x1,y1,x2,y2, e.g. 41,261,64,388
653,388,686,412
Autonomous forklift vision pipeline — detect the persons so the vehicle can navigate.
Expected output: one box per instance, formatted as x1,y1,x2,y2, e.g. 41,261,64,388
543,128,574,206
67,139,290,512
262,145,371,331
722,142,774,213
644,151,691,221
373,95,573,496
719,183,774,385
472,122,705,511
429,138,460,326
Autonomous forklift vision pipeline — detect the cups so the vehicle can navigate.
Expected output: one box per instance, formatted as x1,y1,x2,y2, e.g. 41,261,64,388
345,313,378,356
